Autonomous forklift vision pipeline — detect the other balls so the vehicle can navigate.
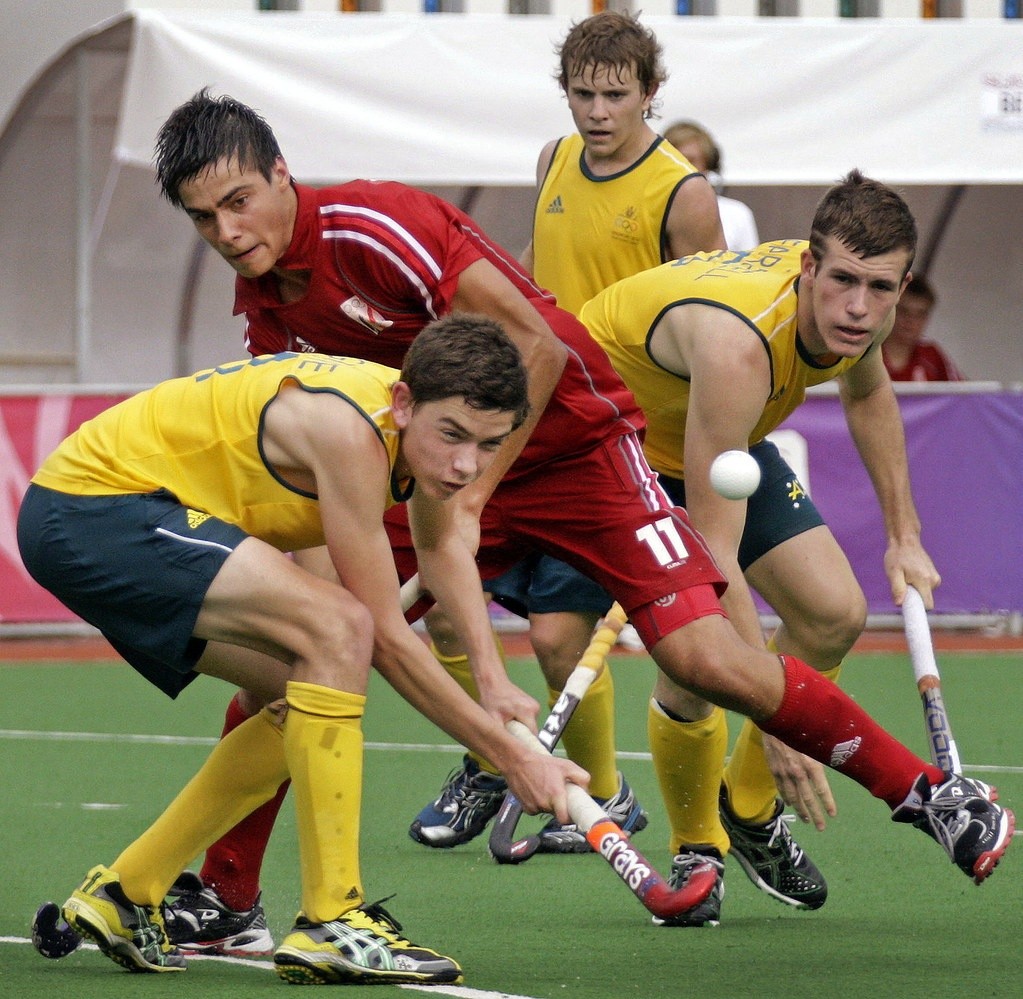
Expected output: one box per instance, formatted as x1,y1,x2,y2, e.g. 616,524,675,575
711,450,762,506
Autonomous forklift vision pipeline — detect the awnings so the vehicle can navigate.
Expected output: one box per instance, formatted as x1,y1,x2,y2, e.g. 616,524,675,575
114,3,1023,193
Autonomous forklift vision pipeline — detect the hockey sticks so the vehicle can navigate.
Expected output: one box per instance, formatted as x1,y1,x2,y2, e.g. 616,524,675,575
893,579,969,781
490,595,630,864
502,719,721,921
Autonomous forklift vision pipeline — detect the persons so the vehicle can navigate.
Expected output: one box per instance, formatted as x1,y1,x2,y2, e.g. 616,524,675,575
881,277,968,385
24,305,598,989
150,84,1019,957
409,3,733,851
657,116,765,255
576,165,942,928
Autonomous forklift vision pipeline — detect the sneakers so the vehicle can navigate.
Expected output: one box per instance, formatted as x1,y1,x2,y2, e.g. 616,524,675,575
654,846,725,927
409,754,510,848
889,769,1016,886
719,780,827,909
273,909,464,986
532,772,647,853
66,863,187,973
160,872,274,956
